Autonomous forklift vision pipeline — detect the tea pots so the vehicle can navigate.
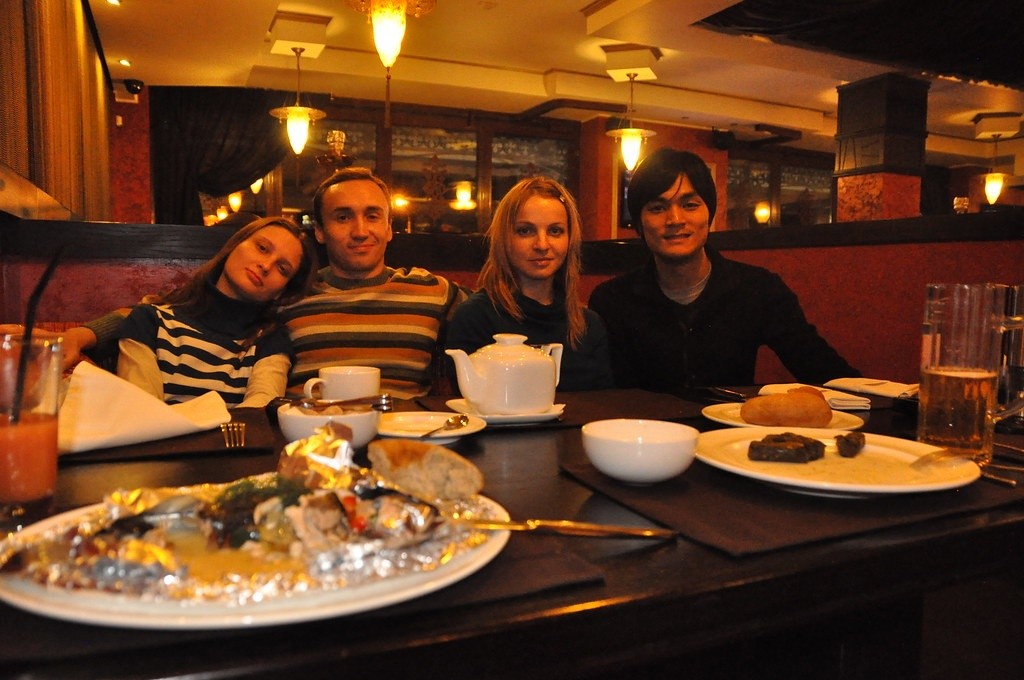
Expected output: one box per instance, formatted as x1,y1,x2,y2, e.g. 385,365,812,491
445,333,563,416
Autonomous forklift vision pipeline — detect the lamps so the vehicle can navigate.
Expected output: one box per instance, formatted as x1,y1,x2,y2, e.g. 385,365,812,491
269,46,327,157
973,133,1014,205
753,192,771,224
345,0,438,130
443,144,478,211
606,74,657,172
204,178,263,226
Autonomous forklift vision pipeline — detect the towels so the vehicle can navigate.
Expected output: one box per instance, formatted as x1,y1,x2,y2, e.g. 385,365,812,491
823,378,919,399
755,383,871,410
31,359,231,456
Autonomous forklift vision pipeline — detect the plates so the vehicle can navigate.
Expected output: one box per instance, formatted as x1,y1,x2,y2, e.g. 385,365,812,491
377,411,487,445
693,427,981,500
701,402,865,431
445,398,564,424
0,484,510,629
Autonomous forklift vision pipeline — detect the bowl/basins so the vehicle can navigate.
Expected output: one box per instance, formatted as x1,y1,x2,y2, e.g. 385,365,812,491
278,399,379,449
581,419,700,485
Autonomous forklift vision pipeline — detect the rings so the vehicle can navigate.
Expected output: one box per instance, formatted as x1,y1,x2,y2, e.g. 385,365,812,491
17,324,22,327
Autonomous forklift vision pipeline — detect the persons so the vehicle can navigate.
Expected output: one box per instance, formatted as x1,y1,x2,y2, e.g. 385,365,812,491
432,173,616,396
1,168,473,401
587,149,866,397
115,215,315,411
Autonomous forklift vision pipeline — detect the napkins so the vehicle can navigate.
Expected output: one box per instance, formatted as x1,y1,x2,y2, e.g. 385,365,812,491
758,383,872,410
822,377,921,398
56,358,232,458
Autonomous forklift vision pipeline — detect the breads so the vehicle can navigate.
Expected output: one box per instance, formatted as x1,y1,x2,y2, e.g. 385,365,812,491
366,438,485,502
739,385,833,427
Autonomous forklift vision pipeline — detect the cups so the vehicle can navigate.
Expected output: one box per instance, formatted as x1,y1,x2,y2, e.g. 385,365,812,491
303,365,381,401
0,332,68,528
916,282,1005,467
997,285,1024,434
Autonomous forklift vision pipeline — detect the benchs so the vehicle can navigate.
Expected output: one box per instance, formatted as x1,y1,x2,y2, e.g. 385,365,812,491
0,207,1024,406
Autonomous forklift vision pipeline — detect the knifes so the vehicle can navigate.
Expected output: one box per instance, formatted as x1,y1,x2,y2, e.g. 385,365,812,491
436,517,680,538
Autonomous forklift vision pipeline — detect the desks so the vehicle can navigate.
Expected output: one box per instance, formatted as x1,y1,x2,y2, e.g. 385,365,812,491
0,384,1024,680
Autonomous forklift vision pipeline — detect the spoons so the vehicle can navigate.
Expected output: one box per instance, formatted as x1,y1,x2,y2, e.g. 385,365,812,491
418,415,469,441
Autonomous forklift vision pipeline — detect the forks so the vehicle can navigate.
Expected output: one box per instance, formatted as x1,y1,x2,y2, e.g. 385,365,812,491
216,422,246,448
909,448,1024,473
